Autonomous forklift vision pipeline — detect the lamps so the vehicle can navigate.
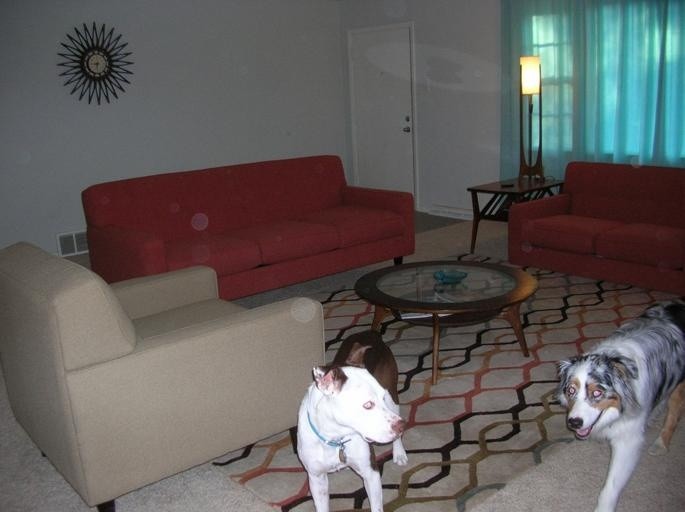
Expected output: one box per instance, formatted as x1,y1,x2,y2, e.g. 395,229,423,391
519,56,546,184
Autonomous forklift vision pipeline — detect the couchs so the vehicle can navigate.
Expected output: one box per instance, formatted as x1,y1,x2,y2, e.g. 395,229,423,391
506,160,685,299
0,238,328,509
79,154,417,303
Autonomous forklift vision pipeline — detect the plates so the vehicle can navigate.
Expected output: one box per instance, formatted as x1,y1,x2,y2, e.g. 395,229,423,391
433,270,469,283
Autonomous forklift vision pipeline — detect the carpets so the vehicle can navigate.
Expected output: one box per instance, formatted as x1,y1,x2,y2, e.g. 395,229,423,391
207,251,685,511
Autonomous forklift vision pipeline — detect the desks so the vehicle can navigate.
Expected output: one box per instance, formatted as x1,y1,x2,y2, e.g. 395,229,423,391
464,174,564,256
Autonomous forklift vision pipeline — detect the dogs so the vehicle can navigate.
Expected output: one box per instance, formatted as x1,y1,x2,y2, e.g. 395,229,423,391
556,297,685,512
296,327,410,512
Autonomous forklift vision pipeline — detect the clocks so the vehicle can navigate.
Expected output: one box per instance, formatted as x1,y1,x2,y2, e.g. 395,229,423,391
56,21,134,107
